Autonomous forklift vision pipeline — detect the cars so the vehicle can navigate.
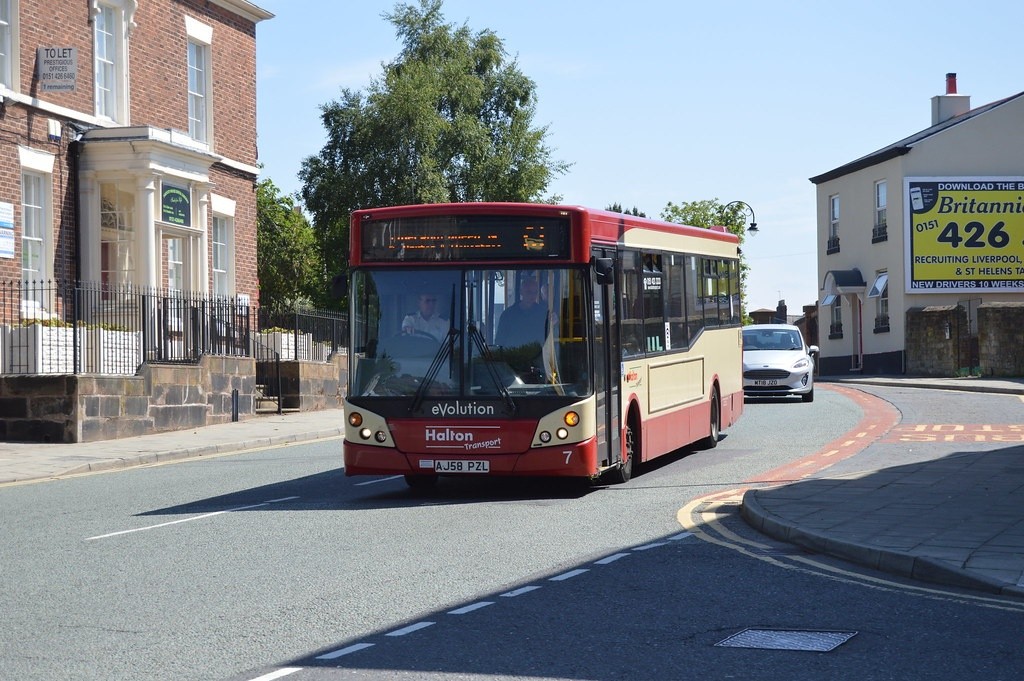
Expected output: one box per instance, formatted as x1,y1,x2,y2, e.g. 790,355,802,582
741,323,820,403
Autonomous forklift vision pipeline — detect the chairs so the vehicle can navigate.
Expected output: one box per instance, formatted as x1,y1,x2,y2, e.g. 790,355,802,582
779,334,794,348
624,290,683,319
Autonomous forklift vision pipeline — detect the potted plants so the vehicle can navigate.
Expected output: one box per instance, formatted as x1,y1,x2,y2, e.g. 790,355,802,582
0,318,143,375
248,325,313,361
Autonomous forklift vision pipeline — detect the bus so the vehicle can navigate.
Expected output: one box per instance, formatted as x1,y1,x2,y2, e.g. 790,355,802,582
330,199,746,487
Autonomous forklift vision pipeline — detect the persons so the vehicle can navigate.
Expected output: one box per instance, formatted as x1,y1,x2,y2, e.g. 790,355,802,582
494,278,558,347
401,291,450,343
540,283,559,313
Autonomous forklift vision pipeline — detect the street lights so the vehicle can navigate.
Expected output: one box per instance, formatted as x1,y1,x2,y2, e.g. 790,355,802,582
716,199,760,236
